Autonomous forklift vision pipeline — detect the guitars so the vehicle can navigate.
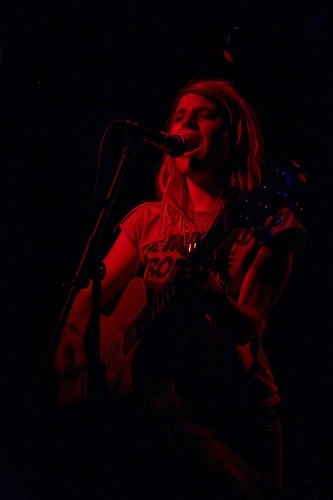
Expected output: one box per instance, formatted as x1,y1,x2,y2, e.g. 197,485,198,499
62,174,294,406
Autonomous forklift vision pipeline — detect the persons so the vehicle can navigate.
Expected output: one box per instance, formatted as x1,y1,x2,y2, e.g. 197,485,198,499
54,79,307,500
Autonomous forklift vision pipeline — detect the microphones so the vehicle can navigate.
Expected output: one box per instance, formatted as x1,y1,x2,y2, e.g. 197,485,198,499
126,119,185,158
